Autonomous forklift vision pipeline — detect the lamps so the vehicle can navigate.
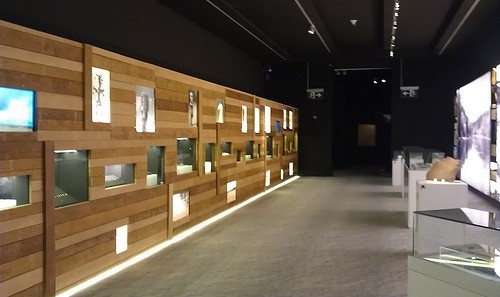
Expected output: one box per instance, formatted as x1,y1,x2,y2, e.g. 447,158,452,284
307,27,315,34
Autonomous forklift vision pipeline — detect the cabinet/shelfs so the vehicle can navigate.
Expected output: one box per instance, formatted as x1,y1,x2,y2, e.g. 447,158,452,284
406,207,500,297
392,155,404,199
408,168,428,228
415,180,468,255
